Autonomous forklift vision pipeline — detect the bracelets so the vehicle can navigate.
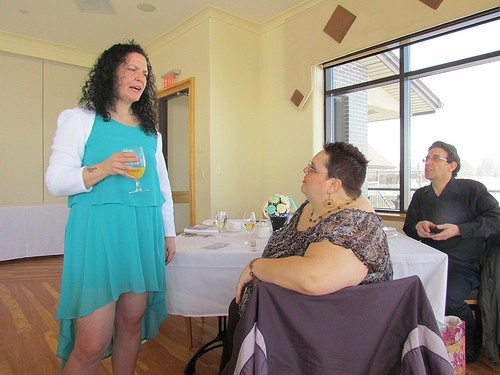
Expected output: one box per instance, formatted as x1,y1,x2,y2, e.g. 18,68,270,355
248,258,260,278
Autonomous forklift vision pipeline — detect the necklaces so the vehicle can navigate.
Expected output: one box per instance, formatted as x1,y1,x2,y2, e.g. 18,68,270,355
308,197,358,222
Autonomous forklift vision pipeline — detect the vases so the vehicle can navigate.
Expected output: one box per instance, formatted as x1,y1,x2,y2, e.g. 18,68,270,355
269,215,288,232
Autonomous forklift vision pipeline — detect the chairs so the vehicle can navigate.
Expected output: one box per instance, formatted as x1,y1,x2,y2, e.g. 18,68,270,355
221,275,454,375
464,236,500,348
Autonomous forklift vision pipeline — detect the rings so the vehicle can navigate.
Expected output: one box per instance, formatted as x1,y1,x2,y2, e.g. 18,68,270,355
235,284,239,289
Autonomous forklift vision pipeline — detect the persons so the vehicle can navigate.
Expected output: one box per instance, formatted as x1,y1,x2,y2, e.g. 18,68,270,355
402,142,500,360
218,142,394,375
44,38,176,375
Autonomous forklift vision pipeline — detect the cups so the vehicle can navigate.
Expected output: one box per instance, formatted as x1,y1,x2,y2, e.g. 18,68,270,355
254,220,271,238
383,227,396,236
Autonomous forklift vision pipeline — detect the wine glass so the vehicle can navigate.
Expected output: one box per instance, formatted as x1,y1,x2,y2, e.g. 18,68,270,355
122,146,149,195
242,212,256,244
216,212,228,237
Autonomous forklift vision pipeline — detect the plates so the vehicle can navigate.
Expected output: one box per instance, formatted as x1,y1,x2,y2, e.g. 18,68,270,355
224,224,247,232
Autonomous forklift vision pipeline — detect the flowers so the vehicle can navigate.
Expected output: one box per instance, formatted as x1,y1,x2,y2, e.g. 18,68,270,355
263,194,297,214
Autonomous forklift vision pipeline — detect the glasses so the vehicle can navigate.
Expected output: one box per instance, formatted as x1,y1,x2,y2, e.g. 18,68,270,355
422,154,448,163
308,159,337,178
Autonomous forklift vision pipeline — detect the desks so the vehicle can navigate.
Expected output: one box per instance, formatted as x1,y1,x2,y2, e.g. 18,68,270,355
165,218,449,348
